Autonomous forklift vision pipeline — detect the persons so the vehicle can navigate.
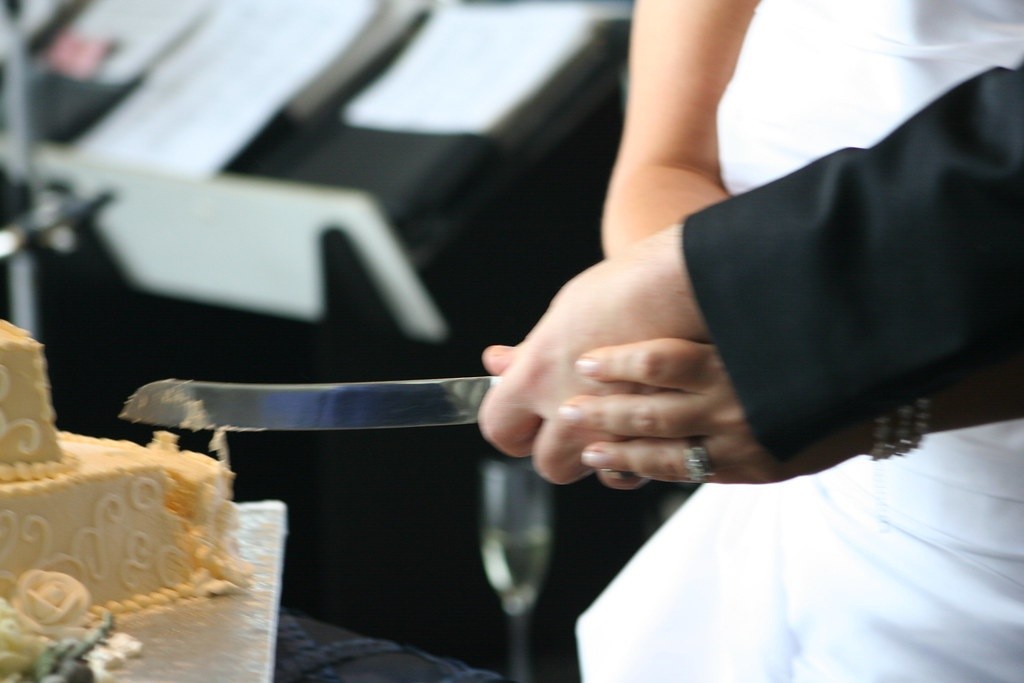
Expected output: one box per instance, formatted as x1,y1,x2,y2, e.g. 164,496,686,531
470,64,1024,485
571,0,1022,683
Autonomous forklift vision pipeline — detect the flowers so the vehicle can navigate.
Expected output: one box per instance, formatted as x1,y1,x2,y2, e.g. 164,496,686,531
1,567,144,683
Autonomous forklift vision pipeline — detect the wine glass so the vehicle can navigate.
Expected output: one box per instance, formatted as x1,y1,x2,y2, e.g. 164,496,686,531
480,458,555,682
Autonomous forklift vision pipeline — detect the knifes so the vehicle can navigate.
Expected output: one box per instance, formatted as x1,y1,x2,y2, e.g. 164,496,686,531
116,374,498,439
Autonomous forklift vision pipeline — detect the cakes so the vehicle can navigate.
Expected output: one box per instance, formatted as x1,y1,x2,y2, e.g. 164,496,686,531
0,306,248,683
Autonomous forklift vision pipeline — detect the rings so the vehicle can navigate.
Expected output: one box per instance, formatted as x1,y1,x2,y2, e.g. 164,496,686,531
687,437,709,484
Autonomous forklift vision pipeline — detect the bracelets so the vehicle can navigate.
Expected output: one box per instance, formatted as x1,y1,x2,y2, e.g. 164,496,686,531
870,396,929,464
